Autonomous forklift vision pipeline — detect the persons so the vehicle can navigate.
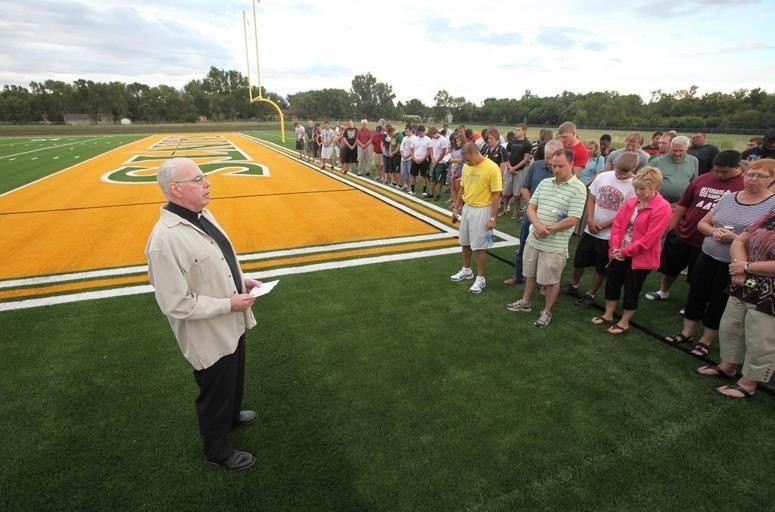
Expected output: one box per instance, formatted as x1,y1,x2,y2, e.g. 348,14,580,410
450,142,503,294
142,157,265,471
504,121,773,399
294,117,531,223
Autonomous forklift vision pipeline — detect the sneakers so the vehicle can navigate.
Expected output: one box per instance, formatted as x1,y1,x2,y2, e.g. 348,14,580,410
320,165,453,204
450,269,598,330
498,206,526,220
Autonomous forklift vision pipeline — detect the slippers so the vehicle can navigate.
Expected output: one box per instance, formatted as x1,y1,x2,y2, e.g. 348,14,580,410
591,291,754,399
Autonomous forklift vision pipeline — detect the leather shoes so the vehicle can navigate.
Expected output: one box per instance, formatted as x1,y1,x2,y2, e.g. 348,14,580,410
206,449,257,470
238,410,259,427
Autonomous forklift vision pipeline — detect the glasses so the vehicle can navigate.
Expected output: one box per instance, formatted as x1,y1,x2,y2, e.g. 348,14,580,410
744,172,774,180
174,174,207,185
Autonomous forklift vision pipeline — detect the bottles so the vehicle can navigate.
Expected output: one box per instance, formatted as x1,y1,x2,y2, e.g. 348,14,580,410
552,209,567,219
485,229,493,248
